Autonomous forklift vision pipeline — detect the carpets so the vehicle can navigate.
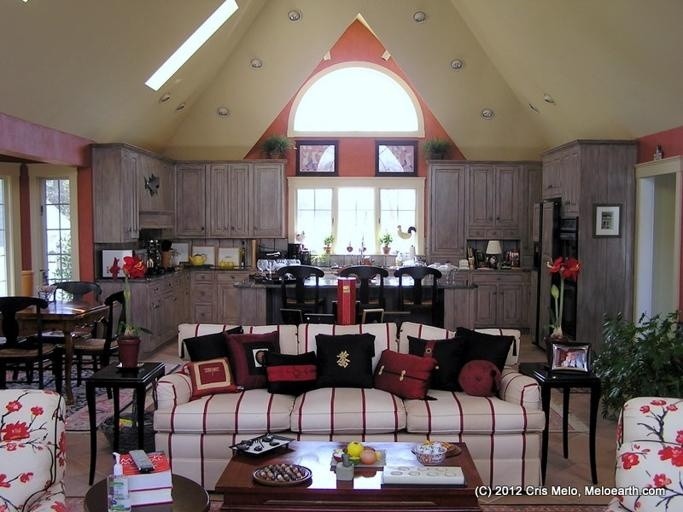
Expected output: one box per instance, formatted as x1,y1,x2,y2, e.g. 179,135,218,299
0,354,182,433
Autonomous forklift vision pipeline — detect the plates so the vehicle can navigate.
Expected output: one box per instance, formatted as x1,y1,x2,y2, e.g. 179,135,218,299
250,463,312,486
412,441,461,458
328,448,388,469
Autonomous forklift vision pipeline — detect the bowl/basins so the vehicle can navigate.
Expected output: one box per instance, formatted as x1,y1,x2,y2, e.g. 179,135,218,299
414,444,447,467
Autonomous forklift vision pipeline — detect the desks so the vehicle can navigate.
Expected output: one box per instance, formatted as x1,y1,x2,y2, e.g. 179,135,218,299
82,472,211,512
517,359,602,486
233,273,477,328
84,361,165,485
214,440,486,511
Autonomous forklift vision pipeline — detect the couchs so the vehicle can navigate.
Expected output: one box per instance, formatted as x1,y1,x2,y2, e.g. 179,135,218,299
151,321,547,496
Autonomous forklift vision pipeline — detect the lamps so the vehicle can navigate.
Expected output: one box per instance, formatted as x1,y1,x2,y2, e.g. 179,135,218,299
484,240,502,270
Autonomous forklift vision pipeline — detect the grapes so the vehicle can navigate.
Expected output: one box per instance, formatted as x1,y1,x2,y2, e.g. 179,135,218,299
261,464,301,482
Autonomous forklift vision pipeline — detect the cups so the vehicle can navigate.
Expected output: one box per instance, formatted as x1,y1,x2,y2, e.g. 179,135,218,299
219,262,233,267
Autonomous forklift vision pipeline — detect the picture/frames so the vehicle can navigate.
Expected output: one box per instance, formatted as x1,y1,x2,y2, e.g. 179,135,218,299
547,338,593,378
374,143,418,177
293,139,339,177
592,202,624,239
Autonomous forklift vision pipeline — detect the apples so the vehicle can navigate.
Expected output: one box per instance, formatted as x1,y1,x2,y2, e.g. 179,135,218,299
361,450,376,464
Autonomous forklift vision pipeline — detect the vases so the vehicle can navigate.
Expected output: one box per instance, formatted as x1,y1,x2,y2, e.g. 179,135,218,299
116,337,142,368
546,337,569,364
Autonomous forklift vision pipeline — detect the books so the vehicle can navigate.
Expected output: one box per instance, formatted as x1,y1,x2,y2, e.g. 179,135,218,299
119,450,177,507
382,465,464,485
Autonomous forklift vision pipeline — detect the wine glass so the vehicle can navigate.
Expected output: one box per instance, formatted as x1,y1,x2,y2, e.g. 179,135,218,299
256,258,302,280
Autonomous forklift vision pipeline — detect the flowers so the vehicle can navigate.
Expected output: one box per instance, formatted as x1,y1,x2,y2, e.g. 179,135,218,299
543,254,583,337
106,253,156,336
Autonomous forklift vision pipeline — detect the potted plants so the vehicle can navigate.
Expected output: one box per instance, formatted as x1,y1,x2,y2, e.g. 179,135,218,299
378,233,394,254
416,134,453,160
322,233,335,254
259,130,297,160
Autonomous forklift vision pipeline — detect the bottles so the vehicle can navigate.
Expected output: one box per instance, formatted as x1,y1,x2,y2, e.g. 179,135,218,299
396,252,402,266
508,248,519,266
409,245,415,258
302,247,311,265
239,247,245,267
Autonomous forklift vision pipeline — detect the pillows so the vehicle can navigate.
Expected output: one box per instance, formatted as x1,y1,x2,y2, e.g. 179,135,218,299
176,322,516,403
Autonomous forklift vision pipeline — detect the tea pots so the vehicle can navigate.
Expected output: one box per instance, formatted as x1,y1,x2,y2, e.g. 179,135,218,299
188,253,208,265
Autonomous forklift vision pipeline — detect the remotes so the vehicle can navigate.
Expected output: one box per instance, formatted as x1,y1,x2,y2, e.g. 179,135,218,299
129,449,155,472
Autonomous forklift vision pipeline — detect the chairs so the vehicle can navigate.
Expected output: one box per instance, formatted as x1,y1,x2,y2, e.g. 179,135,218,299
392,265,441,321
0,386,67,511
0,295,62,395
339,266,389,322
605,394,682,511
275,264,326,322
72,288,126,387
26,281,102,379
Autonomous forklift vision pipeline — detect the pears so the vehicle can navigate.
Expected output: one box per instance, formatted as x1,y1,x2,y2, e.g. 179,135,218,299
347,441,363,457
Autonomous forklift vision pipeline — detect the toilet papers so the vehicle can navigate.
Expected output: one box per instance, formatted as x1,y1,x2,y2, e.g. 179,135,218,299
106,452,132,512
409,244,415,257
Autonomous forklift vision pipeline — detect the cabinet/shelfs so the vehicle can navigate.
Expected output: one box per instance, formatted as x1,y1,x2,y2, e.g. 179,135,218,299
535,200,555,354
189,245,216,267
191,272,216,319
104,278,159,355
216,269,267,326
89,141,139,245
252,160,290,239
423,162,465,264
215,246,241,269
558,143,577,218
204,162,251,239
540,149,562,197
471,271,536,328
171,241,192,264
159,272,191,346
172,162,204,239
518,162,541,270
465,163,518,240
